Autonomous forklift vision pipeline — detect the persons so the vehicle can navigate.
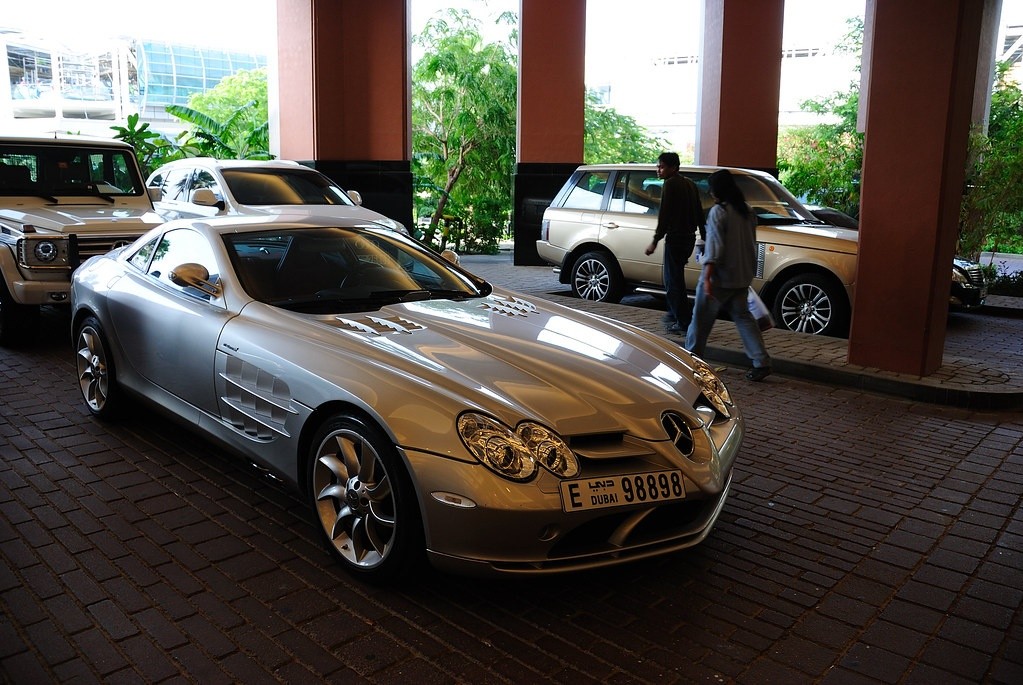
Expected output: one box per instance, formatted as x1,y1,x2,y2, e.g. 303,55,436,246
646,153,706,335
682,169,774,382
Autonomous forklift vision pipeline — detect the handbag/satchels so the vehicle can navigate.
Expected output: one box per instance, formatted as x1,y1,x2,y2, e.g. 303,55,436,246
747,286,774,331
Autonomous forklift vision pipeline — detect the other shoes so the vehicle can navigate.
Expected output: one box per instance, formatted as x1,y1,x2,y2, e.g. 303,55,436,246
746,367,771,381
664,322,686,336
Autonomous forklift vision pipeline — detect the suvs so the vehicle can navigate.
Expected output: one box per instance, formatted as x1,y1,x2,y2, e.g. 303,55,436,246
1,138,168,327
535,163,860,336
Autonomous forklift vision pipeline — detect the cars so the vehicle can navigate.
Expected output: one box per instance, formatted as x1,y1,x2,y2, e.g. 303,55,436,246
802,205,987,316
67,213,747,581
146,157,414,276
